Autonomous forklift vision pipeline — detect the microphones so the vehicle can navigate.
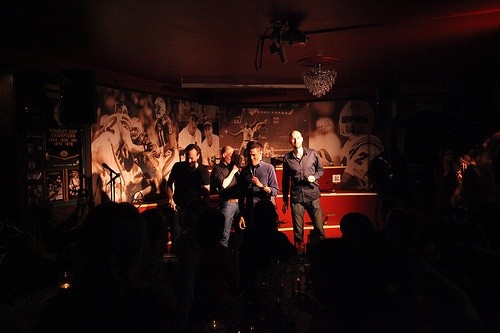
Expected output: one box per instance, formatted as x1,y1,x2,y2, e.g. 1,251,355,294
304,175,320,187
248,163,253,177
102,163,117,175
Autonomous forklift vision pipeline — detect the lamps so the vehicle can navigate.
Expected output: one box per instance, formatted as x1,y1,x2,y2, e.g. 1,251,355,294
301,64,343,98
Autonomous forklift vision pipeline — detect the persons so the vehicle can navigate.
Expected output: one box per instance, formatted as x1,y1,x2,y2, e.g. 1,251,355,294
298,130,500,332
1,197,298,332
90,96,220,204
332,98,384,192
221,117,274,165
283,131,326,242
165,142,280,247
